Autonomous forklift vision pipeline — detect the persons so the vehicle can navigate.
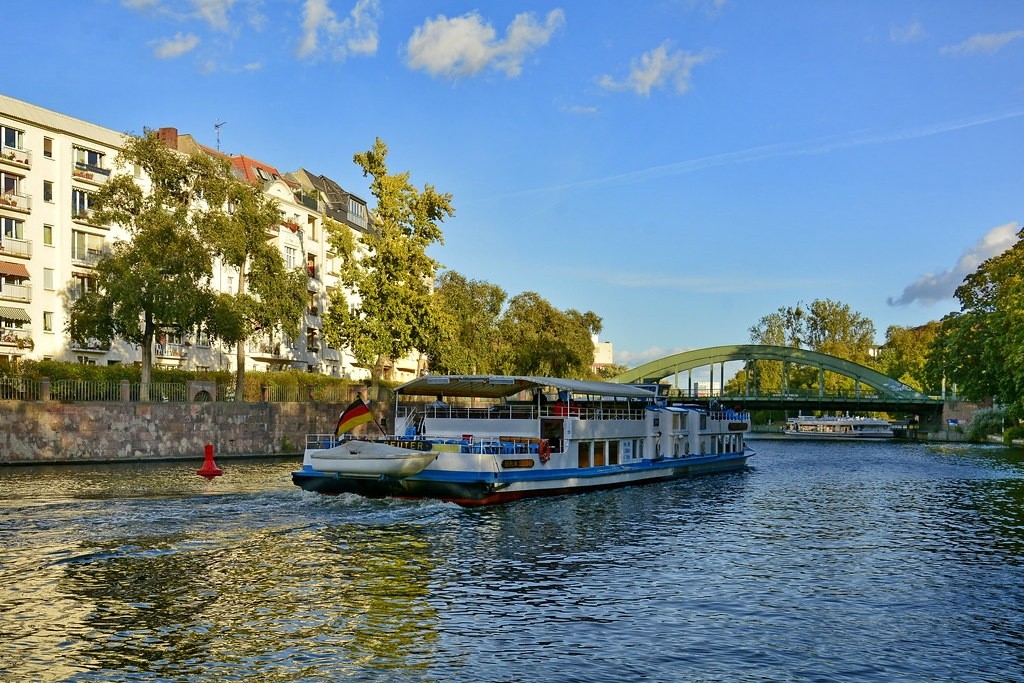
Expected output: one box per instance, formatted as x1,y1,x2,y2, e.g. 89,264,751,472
533,388,581,416
427,394,446,409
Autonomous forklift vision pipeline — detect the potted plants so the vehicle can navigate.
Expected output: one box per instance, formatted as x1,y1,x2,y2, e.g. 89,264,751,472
11,201,17,207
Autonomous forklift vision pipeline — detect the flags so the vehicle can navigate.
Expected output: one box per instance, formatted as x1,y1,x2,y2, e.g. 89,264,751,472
334,398,375,440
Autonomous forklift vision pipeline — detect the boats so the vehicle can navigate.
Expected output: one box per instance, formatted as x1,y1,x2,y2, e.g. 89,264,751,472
784,415,900,441
290,374,755,504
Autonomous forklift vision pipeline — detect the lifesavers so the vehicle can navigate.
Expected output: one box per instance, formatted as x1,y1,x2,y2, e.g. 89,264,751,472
539,440,550,462
